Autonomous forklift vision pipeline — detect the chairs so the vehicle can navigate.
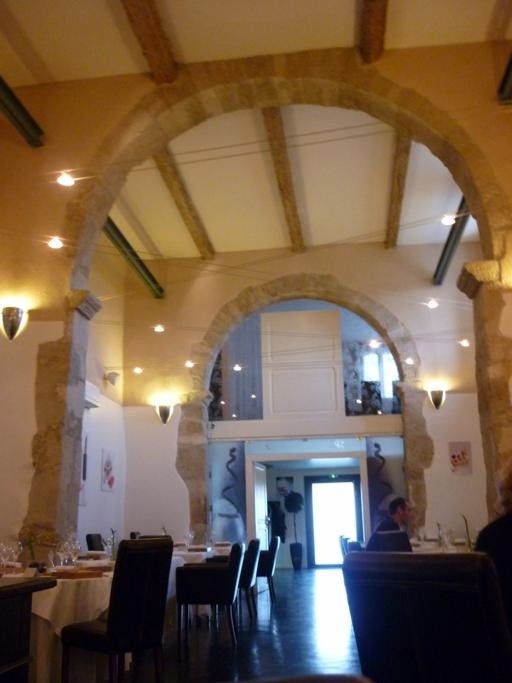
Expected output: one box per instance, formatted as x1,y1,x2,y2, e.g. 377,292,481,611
339,535,512,683
58,531,280,683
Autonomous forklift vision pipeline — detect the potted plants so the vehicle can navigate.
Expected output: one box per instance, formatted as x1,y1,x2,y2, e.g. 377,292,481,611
283,491,304,571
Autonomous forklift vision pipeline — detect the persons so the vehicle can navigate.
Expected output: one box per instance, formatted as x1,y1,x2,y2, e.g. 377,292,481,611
474,453,512,604
361,495,420,553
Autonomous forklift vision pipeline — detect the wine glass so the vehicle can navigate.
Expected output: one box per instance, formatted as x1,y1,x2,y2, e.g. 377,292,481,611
0,541,22,578
55,541,81,572
